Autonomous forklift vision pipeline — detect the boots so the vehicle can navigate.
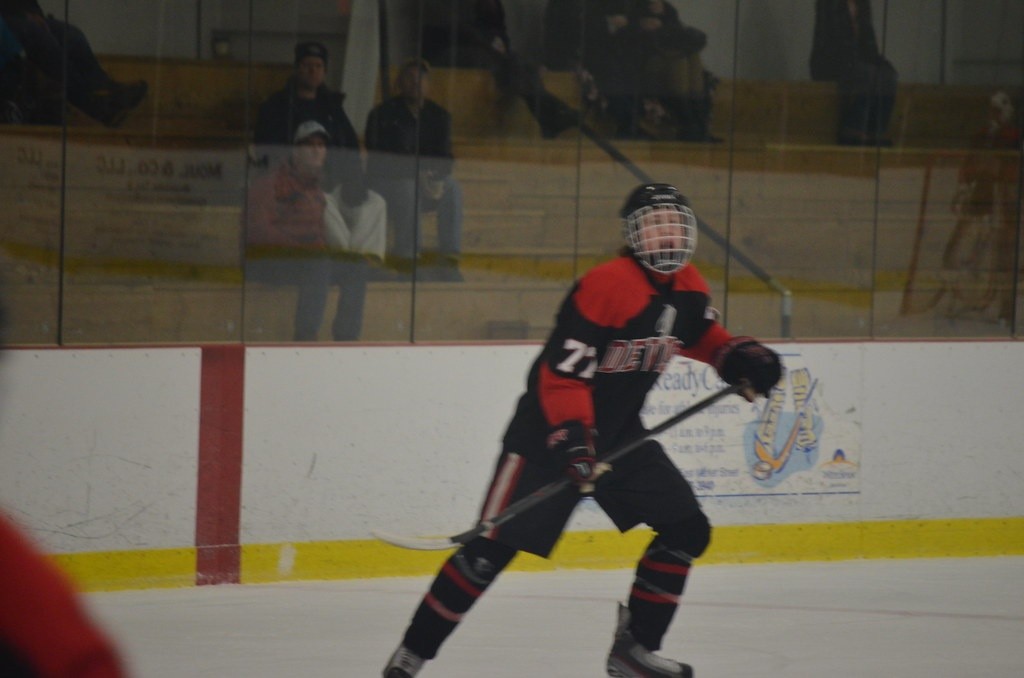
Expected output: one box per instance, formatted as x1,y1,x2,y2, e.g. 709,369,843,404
528,89,724,142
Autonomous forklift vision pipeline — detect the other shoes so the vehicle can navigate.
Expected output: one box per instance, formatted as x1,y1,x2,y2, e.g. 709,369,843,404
403,258,464,283
838,126,894,147
95,80,148,127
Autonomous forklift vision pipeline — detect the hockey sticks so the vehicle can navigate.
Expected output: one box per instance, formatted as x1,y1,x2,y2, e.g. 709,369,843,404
368,385,746,551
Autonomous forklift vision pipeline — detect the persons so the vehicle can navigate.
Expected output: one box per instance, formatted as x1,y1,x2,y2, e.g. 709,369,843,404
0,0,150,130
361,57,465,283
808,0,900,149
540,0,630,126
379,182,785,678
252,39,390,283
596,0,725,150
417,0,582,143
247,121,371,340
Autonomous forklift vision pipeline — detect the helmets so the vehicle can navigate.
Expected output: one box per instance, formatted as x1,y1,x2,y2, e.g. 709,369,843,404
619,182,690,217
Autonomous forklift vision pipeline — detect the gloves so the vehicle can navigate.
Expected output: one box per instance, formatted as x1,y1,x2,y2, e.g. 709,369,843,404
544,420,615,501
714,334,786,403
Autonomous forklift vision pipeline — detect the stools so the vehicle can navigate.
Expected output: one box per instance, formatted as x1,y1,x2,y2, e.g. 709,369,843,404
2,52,1024,340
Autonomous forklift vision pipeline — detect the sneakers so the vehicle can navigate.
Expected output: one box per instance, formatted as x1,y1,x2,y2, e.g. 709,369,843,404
381,640,423,678
605,601,693,678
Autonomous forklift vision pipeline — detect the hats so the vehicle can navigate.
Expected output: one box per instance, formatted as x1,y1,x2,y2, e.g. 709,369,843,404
399,57,431,74
295,41,328,67
293,120,330,142
